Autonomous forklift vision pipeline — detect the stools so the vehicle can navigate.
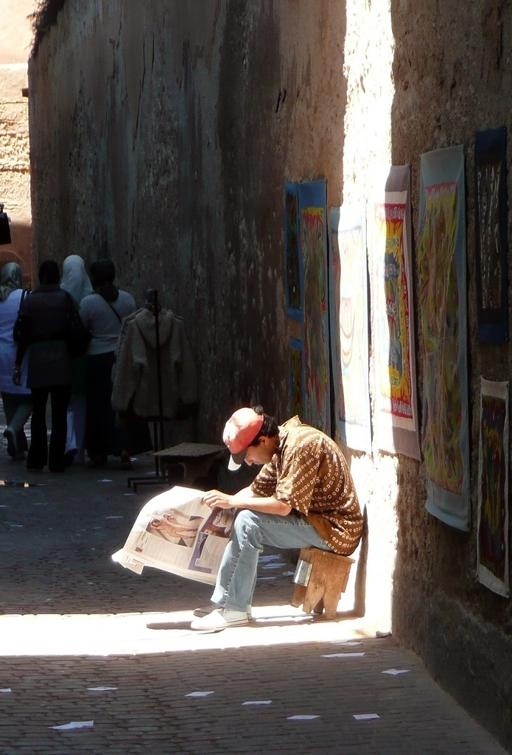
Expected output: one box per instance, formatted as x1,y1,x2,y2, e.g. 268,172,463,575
291,545,356,618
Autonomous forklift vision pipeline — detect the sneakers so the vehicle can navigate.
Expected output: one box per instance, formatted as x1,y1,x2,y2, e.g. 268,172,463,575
190,601,249,632
3,427,19,461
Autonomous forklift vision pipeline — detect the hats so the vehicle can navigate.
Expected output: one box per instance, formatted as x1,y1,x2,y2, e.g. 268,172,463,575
220,406,265,472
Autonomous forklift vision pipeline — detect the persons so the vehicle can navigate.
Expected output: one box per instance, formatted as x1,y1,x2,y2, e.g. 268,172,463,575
0,240,153,473
190,405,365,631
